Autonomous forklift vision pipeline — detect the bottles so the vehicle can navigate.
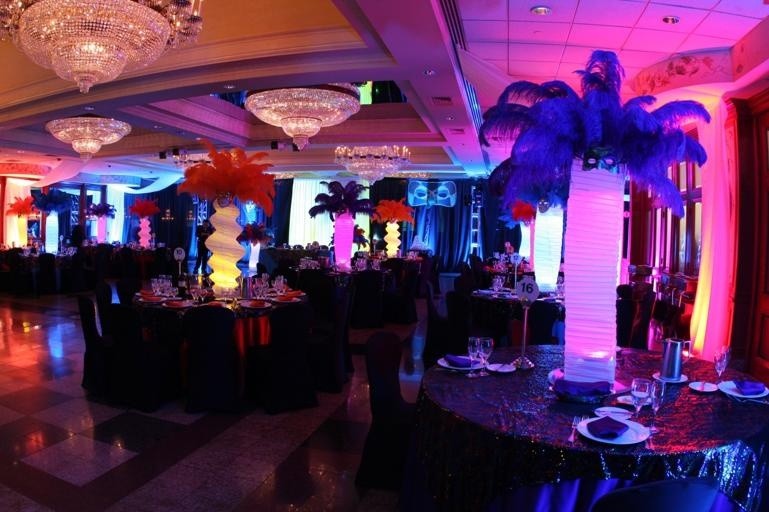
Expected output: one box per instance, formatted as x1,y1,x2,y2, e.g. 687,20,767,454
164,206,172,221
187,207,193,222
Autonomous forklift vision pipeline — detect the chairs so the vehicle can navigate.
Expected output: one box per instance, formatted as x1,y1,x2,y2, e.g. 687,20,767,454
78,241,444,416
418,249,699,350
355,329,422,496
593,475,721,511
1,246,77,300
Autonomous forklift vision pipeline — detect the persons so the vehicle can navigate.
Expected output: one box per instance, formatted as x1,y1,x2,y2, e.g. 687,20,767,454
194,219,212,273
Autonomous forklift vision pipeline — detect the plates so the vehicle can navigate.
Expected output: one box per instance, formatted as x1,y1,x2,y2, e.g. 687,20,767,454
574,416,650,446
688,381,719,393
615,395,655,408
436,355,490,371
592,406,634,420
485,363,519,374
717,378,768,399
651,371,689,383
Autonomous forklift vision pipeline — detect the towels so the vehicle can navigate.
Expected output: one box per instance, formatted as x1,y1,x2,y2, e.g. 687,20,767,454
732,380,766,397
443,352,480,367
588,416,630,440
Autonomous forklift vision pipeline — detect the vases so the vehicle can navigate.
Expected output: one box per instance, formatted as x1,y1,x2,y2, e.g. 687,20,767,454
383,220,402,258
559,154,633,381
334,207,354,271
518,222,532,264
138,216,152,249
204,193,247,296
532,199,564,294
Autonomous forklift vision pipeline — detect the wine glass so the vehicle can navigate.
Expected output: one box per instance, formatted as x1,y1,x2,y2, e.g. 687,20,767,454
1,232,175,258
722,344,733,381
473,241,565,302
466,335,482,379
268,236,433,277
135,272,306,308
630,377,652,421
649,380,667,434
714,351,726,385
479,337,495,377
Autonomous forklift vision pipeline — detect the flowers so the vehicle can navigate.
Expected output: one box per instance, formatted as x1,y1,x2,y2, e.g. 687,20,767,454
370,198,416,228
175,138,278,218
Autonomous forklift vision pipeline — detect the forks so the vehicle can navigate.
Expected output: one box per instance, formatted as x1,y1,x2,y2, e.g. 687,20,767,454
727,394,769,406
581,413,590,422
567,415,581,444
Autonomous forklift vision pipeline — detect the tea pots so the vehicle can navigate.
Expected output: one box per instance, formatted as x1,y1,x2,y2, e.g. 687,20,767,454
659,337,693,381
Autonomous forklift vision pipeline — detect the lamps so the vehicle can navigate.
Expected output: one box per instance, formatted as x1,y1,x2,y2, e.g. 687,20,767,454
46,118,132,160
243,82,360,152
0,0,204,96
332,146,412,183
